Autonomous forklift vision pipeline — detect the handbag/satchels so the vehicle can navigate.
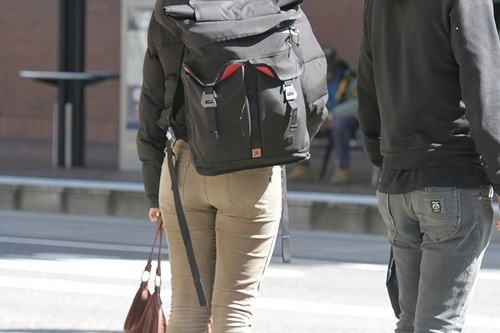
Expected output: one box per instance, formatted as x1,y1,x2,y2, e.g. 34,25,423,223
385,250,403,319
122,214,167,333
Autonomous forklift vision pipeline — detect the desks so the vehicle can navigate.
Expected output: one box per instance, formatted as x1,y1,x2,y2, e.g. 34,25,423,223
20,68,121,166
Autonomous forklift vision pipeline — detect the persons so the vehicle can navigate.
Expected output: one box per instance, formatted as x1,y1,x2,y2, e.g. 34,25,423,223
136,0,329,333
288,44,358,184
356,0,500,332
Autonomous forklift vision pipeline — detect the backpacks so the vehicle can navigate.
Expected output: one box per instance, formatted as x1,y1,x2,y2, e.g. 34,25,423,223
162,0,314,177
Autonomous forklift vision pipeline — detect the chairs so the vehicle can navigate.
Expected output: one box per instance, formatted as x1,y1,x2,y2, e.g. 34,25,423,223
308,130,341,182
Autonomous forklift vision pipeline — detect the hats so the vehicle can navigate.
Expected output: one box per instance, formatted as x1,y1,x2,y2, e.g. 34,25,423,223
321,47,336,61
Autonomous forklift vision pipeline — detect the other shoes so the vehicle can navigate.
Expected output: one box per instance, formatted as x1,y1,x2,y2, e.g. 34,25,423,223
285,165,311,182
330,174,349,186
